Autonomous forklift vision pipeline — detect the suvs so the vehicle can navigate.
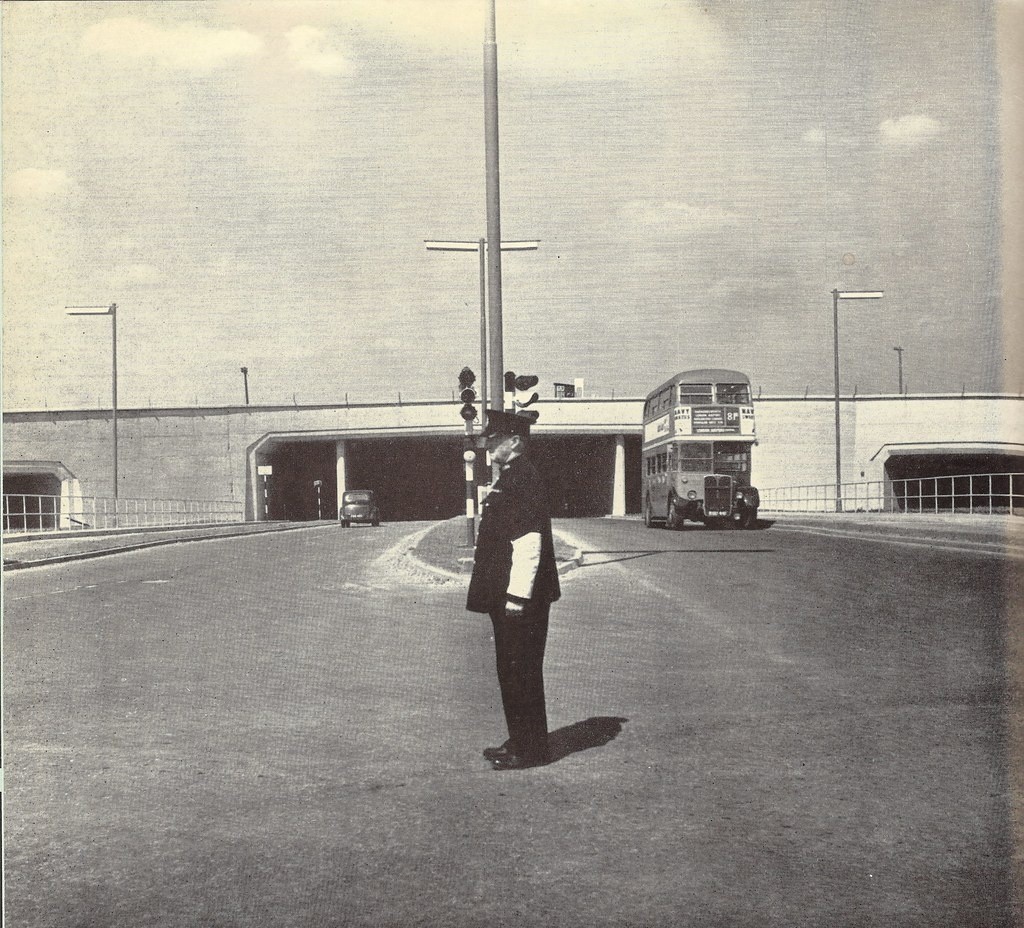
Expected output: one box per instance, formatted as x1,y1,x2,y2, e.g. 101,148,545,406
340,488,381,528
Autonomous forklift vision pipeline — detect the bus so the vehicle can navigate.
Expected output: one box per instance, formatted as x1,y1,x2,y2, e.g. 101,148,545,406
640,368,760,531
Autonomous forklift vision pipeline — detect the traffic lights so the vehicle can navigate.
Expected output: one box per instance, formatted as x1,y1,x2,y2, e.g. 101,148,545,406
516,374,539,422
458,365,476,421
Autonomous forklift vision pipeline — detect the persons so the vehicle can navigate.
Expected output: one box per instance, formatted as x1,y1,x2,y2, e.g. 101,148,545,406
465,406,565,773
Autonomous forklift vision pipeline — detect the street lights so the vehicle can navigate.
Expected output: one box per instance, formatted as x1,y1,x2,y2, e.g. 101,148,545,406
828,286,886,515
421,238,539,435
66,302,119,528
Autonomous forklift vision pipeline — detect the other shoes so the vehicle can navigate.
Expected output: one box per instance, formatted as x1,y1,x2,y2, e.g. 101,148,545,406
493,752,550,770
483,747,512,760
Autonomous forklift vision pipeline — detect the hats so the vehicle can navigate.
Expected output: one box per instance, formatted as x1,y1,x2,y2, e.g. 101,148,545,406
478,409,534,437
